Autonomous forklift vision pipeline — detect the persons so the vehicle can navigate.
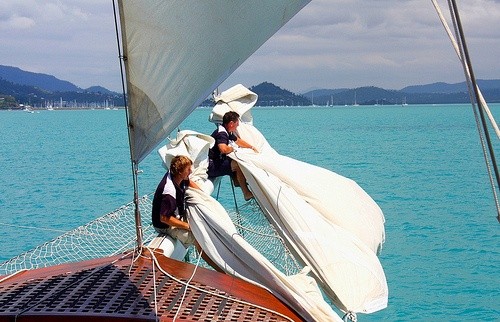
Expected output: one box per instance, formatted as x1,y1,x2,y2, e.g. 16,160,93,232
152,155,226,274
208,111,258,201
